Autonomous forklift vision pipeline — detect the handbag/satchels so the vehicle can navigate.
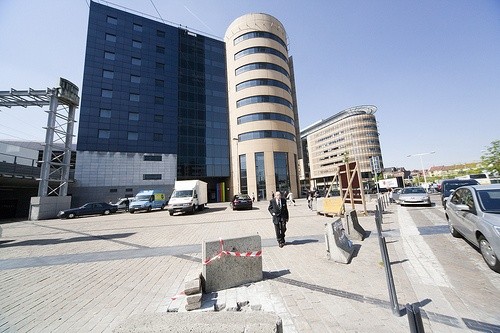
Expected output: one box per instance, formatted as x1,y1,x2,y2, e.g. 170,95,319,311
293,200,295,203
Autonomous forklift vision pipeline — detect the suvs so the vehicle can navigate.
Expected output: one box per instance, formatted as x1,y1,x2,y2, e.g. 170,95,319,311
112,197,135,209
442,179,480,210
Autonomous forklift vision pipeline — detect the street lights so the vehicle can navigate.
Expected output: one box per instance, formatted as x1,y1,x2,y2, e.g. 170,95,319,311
406,151,436,193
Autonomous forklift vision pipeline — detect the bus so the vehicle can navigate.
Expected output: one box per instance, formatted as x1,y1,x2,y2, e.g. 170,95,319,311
455,173,491,185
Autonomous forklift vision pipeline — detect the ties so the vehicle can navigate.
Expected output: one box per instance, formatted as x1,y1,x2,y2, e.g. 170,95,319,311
278,199,280,207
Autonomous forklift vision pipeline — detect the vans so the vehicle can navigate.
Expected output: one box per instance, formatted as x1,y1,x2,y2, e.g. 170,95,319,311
129,190,166,213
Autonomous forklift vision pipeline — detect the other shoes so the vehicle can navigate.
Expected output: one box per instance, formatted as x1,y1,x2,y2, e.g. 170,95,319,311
279,243,284,248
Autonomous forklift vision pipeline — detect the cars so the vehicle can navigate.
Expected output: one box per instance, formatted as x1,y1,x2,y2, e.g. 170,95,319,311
56,202,118,219
392,187,430,206
232,194,252,210
445,184,500,273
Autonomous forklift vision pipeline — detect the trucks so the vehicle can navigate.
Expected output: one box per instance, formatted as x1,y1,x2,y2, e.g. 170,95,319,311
167,179,208,216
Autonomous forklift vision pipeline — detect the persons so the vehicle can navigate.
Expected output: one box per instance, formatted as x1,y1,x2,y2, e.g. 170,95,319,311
307,190,319,208
272,191,274,198
287,190,295,206
268,191,289,247
252,193,255,202
